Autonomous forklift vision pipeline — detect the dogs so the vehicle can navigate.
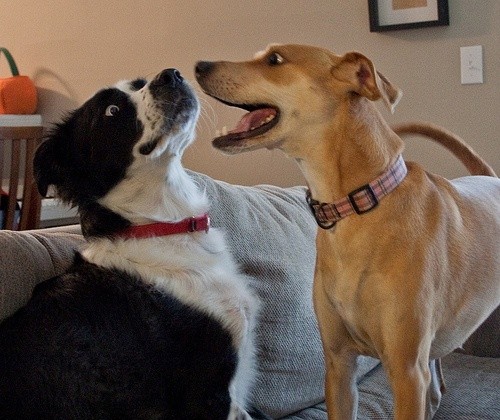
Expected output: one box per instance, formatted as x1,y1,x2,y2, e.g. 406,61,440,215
193,42,499,420
0,67,259,420
